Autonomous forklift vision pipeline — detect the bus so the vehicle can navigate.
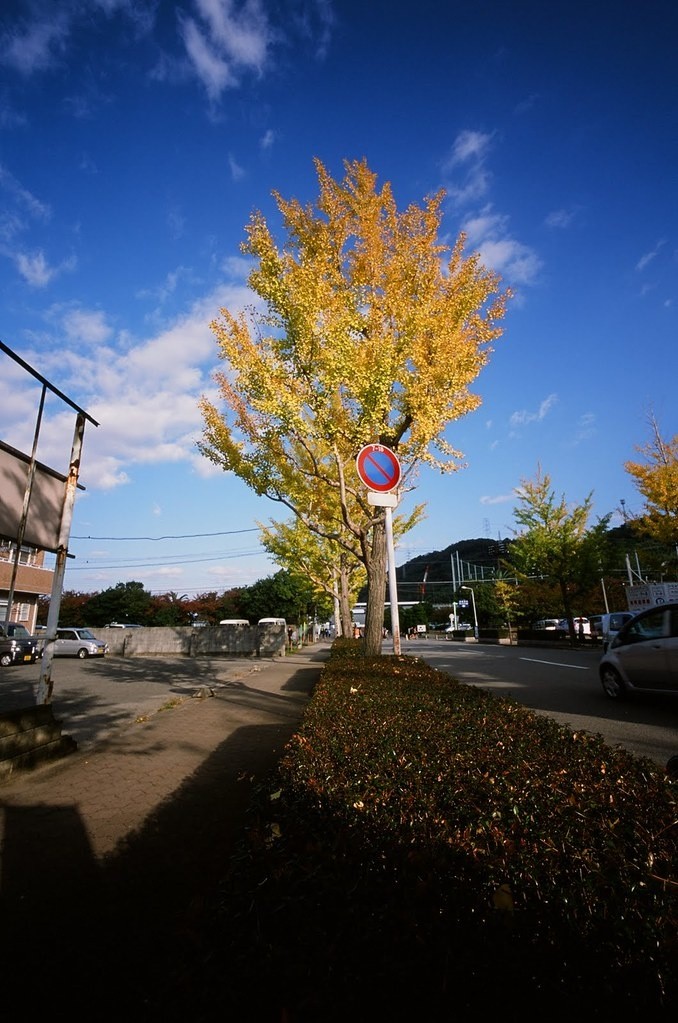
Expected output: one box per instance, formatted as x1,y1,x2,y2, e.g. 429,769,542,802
257,617,287,634
219,619,250,627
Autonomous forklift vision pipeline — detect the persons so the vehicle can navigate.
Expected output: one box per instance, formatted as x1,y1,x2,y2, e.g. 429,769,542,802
325,628,331,637
590,618,595,634
579,616,583,633
407,626,418,638
359,628,363,638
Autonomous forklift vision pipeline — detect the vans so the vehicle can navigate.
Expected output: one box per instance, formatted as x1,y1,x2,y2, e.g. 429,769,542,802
104,621,140,629
0,621,37,667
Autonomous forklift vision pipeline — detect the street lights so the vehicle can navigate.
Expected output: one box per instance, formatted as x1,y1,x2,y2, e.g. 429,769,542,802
461,585,479,639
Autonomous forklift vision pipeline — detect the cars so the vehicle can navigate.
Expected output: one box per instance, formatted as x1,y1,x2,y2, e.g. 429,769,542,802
536,610,662,654
597,601,678,704
39,627,109,659
445,623,471,634
